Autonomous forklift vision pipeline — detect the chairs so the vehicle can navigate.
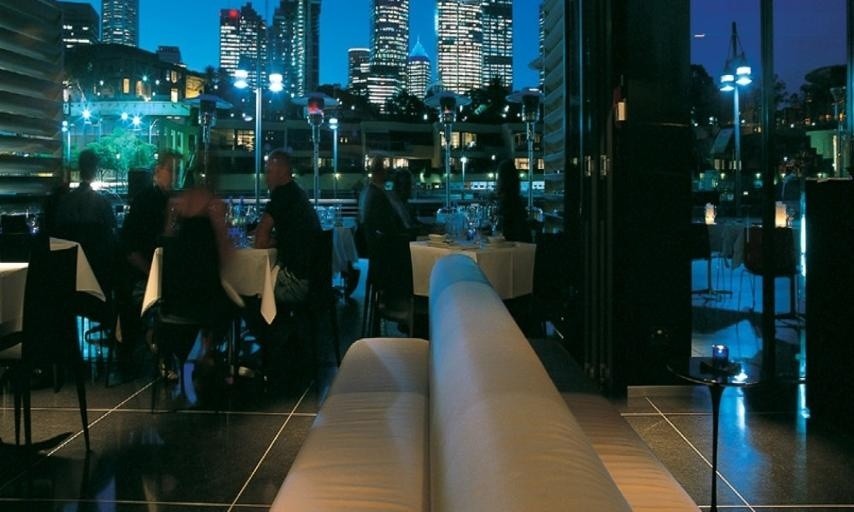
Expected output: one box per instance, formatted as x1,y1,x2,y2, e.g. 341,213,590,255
743,226,797,369
1,212,543,464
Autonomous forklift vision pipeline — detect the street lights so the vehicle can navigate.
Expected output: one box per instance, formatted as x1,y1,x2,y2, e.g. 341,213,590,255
152,152,159,167
64,88,72,119
82,110,90,146
719,22,752,218
290,90,339,207
120,112,141,140
114,149,120,194
507,88,545,215
149,119,160,144
424,91,472,205
235,17,282,215
61,121,70,162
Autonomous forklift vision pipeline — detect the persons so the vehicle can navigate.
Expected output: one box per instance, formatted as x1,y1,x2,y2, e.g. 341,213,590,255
353,153,537,340
46,151,328,379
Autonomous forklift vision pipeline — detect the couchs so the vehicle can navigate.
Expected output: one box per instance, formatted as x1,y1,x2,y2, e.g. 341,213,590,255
267,252,702,512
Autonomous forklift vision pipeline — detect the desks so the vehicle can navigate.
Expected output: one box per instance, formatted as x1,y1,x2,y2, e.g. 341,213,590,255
691,223,736,295
666,358,785,511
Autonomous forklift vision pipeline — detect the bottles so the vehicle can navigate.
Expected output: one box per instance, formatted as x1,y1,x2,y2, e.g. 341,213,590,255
225,193,248,248
114,204,124,233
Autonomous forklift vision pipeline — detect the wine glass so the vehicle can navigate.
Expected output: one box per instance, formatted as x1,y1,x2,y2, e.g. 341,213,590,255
26,207,41,235
464,200,501,248
